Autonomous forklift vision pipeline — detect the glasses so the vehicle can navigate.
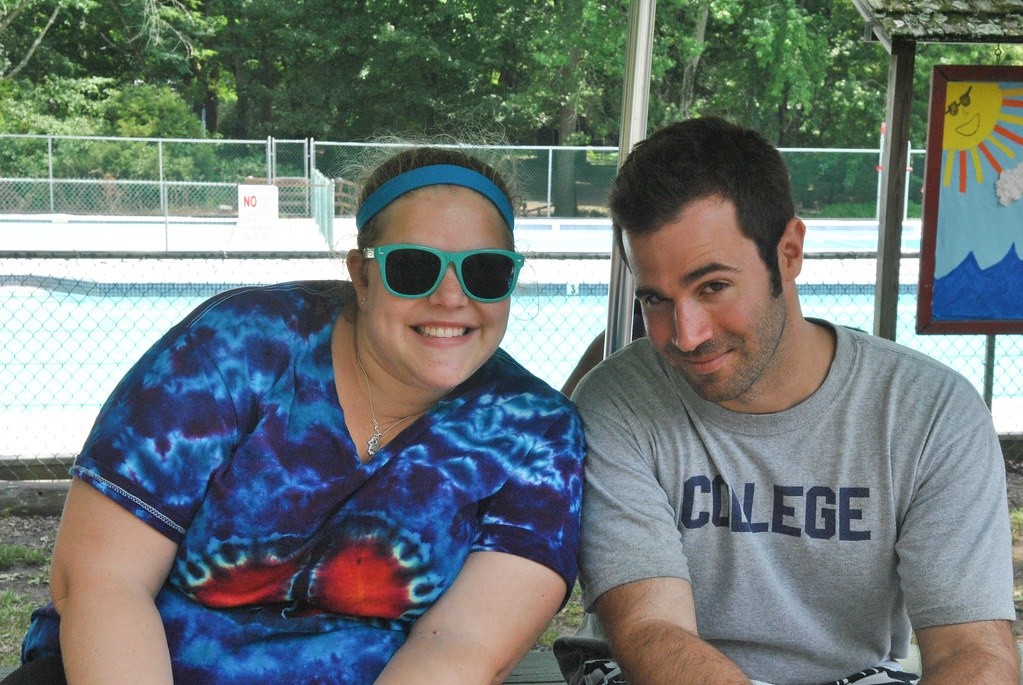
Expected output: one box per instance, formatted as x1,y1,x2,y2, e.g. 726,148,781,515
363,243,525,303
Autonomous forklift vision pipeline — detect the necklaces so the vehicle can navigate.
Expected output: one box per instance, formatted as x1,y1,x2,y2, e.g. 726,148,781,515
354,350,428,456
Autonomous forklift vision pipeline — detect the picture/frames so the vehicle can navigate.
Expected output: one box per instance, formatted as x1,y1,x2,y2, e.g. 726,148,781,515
915,62,1023,336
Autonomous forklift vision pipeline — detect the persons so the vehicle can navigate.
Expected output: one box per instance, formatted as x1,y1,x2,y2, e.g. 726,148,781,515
0,146,589,684
568,111,1022,685
